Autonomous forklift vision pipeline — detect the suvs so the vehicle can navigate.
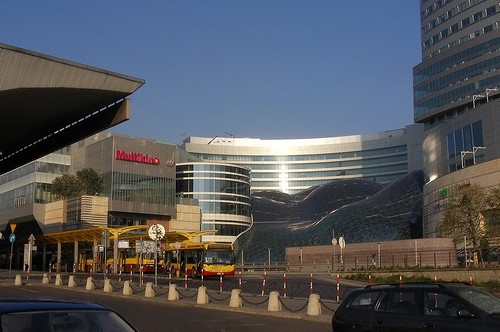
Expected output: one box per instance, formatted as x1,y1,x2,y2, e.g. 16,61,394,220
332,279,500,332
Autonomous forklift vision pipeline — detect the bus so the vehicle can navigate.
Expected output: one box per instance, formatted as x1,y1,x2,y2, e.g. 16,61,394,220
163,242,235,279
79,241,163,274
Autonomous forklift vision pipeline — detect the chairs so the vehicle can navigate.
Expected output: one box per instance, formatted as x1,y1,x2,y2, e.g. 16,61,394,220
446,299,459,317
427,300,443,316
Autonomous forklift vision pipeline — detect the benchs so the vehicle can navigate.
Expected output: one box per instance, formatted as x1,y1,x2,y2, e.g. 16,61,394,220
382,304,416,314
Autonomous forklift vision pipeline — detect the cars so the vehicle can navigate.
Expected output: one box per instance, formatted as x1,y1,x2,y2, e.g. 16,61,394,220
0,295,139,332
